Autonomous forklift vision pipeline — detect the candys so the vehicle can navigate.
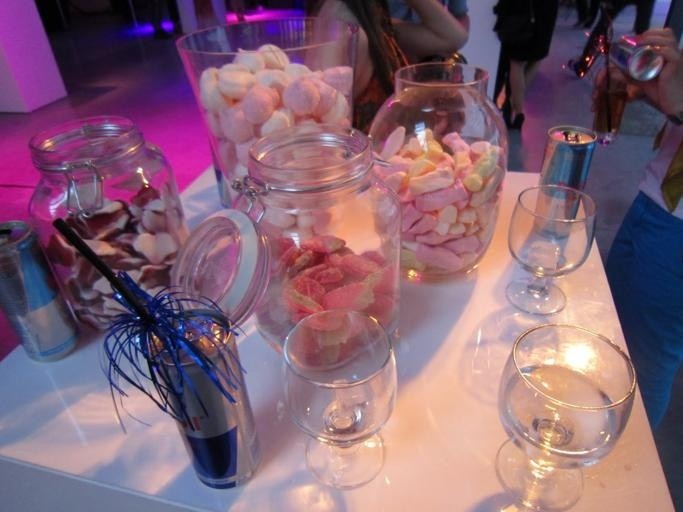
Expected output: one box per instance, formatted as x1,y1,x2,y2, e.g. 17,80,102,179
372,125,509,278
196,44,396,365
49,182,188,333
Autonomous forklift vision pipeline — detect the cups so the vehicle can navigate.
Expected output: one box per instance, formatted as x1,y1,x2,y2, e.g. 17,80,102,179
592,76,629,147
458,305,562,406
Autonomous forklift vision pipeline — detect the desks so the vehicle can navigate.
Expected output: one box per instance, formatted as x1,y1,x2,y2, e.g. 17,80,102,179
1,159,676,511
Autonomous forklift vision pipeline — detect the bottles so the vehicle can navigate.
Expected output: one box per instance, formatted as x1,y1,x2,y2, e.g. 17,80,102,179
171,122,404,372
368,61,508,279
29,114,190,334
175,17,359,209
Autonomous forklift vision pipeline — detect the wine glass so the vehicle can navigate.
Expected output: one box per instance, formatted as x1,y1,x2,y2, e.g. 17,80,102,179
505,183,598,317
492,323,638,511
279,308,400,490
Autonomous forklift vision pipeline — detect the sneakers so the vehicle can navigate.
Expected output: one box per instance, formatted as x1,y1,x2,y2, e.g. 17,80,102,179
568,58,583,79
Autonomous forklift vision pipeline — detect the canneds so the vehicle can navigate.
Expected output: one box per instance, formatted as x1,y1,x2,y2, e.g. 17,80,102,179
142,310,263,489
605,35,666,83
533,126,597,239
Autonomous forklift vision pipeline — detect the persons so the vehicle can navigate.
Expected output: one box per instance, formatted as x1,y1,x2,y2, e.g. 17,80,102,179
495,0,656,132
589,26,683,430
303,0,471,137
143,0,181,38
387,0,472,86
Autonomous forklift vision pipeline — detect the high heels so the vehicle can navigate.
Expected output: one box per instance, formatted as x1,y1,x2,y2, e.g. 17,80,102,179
511,113,525,133
